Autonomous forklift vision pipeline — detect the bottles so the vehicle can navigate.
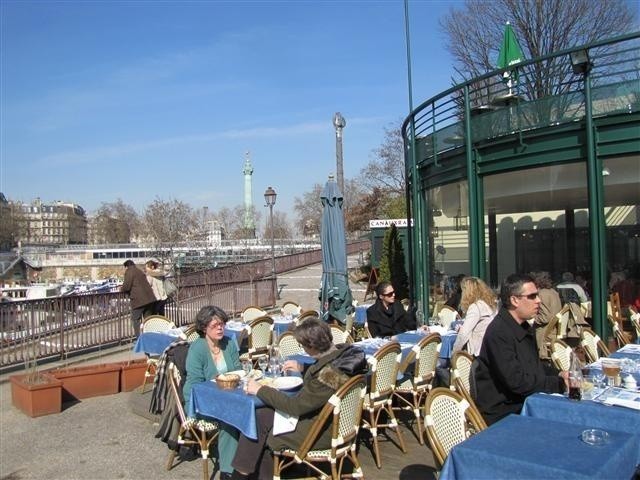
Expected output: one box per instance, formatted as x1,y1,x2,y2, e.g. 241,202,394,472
270,331,281,378
415,301,424,327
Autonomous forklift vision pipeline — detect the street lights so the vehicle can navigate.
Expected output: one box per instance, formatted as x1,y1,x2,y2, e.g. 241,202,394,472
262,186,283,301
563,43,612,362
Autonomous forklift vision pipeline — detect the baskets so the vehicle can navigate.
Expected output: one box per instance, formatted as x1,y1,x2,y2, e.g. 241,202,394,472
214,371,241,391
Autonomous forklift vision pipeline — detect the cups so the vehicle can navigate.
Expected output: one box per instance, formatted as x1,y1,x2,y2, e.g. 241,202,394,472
362,335,392,350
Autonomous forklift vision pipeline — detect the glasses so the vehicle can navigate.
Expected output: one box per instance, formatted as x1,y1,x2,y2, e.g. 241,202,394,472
513,292,539,300
382,291,395,297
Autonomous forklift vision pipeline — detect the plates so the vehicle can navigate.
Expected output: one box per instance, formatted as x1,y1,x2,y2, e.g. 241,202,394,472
257,376,304,391
226,370,262,382
404,318,457,336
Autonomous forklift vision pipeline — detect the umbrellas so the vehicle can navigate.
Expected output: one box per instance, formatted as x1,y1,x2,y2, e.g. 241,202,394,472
493,18,529,96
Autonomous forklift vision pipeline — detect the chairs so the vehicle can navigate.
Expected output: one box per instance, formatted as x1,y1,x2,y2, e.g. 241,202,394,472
362,341,407,469
391,333,442,446
542,299,640,384
142,300,467,394
271,375,367,480
168,362,220,479
450,352,488,430
423,387,485,471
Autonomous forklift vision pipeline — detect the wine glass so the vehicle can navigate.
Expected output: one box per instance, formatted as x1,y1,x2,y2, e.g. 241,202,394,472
258,356,269,379
567,360,637,402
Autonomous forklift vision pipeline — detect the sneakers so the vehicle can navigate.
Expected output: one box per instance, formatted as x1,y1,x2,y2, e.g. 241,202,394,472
222,469,249,480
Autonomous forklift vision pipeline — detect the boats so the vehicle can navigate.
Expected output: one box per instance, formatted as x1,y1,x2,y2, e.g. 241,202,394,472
0,276,127,329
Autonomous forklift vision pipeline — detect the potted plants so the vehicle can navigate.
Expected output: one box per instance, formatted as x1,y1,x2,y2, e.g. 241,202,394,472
117,290,156,392
8,320,65,418
48,302,122,401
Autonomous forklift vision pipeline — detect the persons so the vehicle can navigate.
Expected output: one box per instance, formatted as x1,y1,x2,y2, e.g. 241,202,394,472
366,282,418,339
609,271,633,307
316,172,357,324
556,270,587,303
448,276,500,359
526,268,562,359
474,273,578,428
182,305,243,480
120,260,158,338
143,260,168,317
228,316,369,480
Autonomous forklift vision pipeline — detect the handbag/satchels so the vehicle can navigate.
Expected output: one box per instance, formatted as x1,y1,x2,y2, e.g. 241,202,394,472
163,277,177,299
